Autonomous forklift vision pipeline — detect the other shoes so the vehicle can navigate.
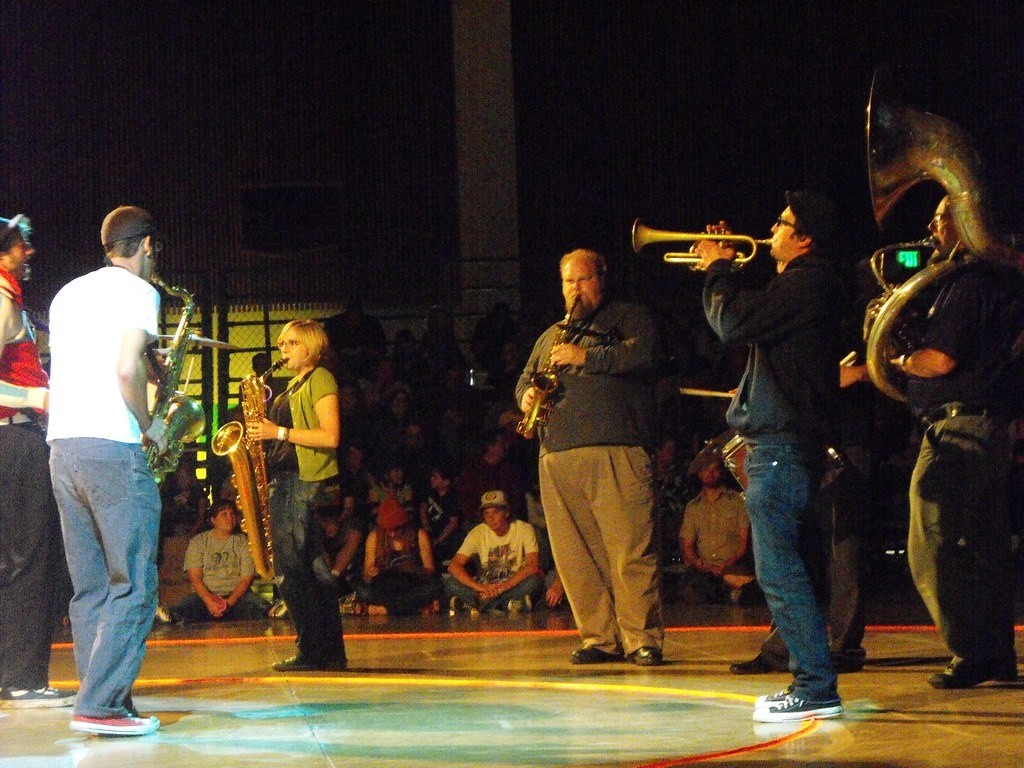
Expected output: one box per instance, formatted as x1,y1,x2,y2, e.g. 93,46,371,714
927,654,985,688
982,661,1019,679
450,596,476,616
511,594,532,613
156,604,172,623
273,654,347,671
272,599,288,618
339,600,363,616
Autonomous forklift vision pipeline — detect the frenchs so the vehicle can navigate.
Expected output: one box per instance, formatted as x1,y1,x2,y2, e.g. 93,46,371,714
864,65,1024,404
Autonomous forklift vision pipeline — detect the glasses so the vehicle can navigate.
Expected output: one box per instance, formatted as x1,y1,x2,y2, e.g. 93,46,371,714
933,212,953,223
276,339,302,350
151,239,163,252
775,216,797,229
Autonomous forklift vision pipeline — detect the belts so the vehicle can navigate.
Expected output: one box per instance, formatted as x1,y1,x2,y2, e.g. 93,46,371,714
0,414,32,426
920,402,1006,430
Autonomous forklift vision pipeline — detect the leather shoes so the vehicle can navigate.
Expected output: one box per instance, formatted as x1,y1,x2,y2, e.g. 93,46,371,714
569,646,625,664
729,650,789,674
831,655,862,673
630,646,663,665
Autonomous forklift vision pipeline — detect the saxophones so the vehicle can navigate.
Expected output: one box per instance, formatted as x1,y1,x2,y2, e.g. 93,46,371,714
212,357,291,581
140,272,206,493
515,293,581,440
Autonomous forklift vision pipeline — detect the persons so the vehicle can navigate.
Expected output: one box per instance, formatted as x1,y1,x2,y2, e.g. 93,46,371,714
316,304,576,617
153,443,294,628
685,189,914,715
514,247,665,665
46,205,175,735
640,275,1024,630
244,320,350,673
862,190,1024,690
0,216,78,701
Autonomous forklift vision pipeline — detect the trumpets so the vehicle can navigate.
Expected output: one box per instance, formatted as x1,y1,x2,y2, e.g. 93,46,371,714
632,219,775,265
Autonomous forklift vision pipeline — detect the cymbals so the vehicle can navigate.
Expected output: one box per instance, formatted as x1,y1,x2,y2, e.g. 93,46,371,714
687,425,739,473
156,333,239,351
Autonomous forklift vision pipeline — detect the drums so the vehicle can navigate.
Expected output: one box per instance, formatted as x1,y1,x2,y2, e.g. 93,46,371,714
720,436,847,492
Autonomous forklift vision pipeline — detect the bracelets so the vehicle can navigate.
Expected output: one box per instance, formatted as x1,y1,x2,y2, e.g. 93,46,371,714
277,426,288,441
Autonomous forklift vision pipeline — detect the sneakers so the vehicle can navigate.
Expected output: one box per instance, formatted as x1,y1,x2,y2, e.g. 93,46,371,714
752,697,844,723
70,714,160,735
1,685,78,709
754,689,791,709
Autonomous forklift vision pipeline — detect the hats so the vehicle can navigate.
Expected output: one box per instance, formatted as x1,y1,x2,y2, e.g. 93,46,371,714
377,492,410,528
0,214,22,240
480,490,508,508
101,204,157,245
